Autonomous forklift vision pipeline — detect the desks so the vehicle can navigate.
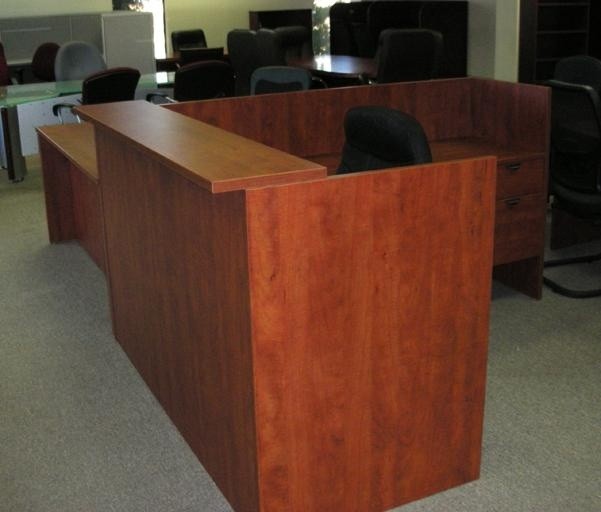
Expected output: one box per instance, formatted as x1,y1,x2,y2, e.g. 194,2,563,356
33,121,107,279
71,76,554,512
0,73,182,181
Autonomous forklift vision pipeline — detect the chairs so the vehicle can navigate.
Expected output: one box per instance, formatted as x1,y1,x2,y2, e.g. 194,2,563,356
54,41,107,81
0,43,19,85
145,23,447,102
332,107,425,174
52,66,142,125
541,76,601,299
30,40,61,83
